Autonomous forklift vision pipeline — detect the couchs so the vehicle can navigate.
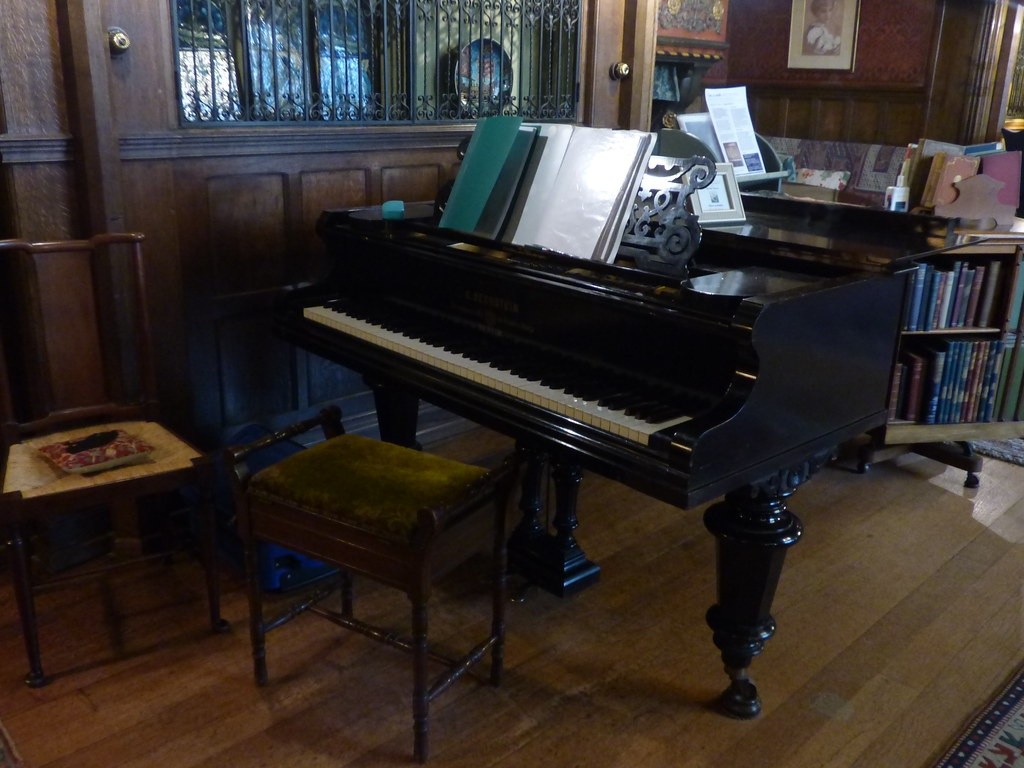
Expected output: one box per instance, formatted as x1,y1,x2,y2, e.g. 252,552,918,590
760,135,908,205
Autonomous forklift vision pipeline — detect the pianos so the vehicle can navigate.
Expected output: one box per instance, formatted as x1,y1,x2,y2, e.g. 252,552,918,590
257,114,923,719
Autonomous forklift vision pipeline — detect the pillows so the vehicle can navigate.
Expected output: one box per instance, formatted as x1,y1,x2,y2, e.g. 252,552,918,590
785,167,851,192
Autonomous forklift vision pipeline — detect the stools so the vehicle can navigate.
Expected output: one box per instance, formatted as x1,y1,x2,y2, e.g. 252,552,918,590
227,403,525,764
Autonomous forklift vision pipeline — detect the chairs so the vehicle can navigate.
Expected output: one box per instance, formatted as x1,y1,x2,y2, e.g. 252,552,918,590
0,230,225,695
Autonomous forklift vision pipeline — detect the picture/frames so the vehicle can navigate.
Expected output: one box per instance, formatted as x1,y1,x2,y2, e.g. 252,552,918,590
786,0,862,73
679,163,747,224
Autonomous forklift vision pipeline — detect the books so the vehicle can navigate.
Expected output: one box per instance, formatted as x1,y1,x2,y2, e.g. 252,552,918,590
901,254,1002,331
888,334,1005,424
438,116,658,264
905,138,1022,208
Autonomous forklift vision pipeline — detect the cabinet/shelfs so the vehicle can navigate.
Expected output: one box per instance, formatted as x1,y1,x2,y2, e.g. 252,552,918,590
855,231,1024,489
673,110,789,192
